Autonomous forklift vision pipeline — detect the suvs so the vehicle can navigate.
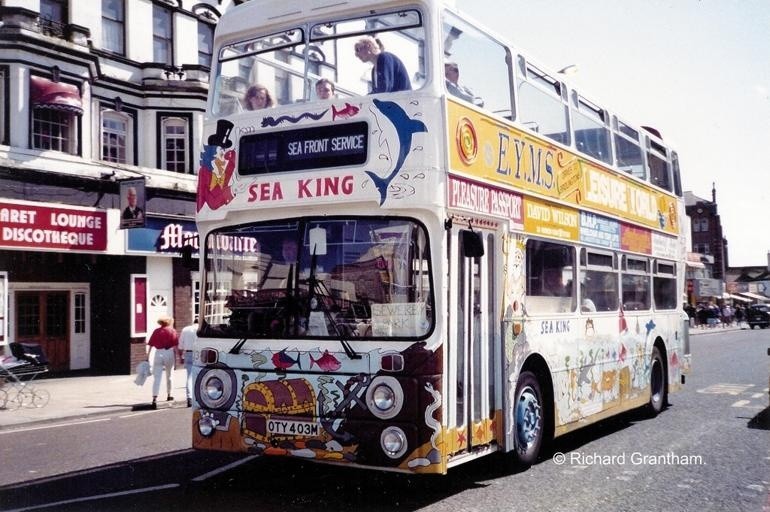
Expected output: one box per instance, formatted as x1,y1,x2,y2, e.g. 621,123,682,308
743,302,770,330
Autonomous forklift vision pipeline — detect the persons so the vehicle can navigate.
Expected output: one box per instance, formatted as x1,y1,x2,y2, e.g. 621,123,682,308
315,78,335,100
565,280,598,311
354,36,414,94
444,61,475,103
685,297,748,330
243,85,275,111
178,312,200,407
145,316,180,411
244,238,304,337
122,185,145,219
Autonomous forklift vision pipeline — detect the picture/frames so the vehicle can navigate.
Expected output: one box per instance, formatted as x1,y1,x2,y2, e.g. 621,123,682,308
117,176,147,231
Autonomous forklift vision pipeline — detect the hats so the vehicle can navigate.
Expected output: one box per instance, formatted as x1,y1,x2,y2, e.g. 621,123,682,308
156,315,174,326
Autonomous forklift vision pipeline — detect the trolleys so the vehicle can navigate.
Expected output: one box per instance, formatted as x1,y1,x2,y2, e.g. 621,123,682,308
0,359,51,413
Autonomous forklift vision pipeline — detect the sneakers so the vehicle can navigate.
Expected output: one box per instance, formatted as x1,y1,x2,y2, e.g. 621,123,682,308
167,396,174,401
187,400,193,407
152,401,157,410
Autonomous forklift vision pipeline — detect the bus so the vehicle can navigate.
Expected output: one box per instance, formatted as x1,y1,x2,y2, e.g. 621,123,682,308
153,1,690,480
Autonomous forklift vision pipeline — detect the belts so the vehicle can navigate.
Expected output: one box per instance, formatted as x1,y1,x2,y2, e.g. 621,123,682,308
186,350,192,352
165,347,171,350
